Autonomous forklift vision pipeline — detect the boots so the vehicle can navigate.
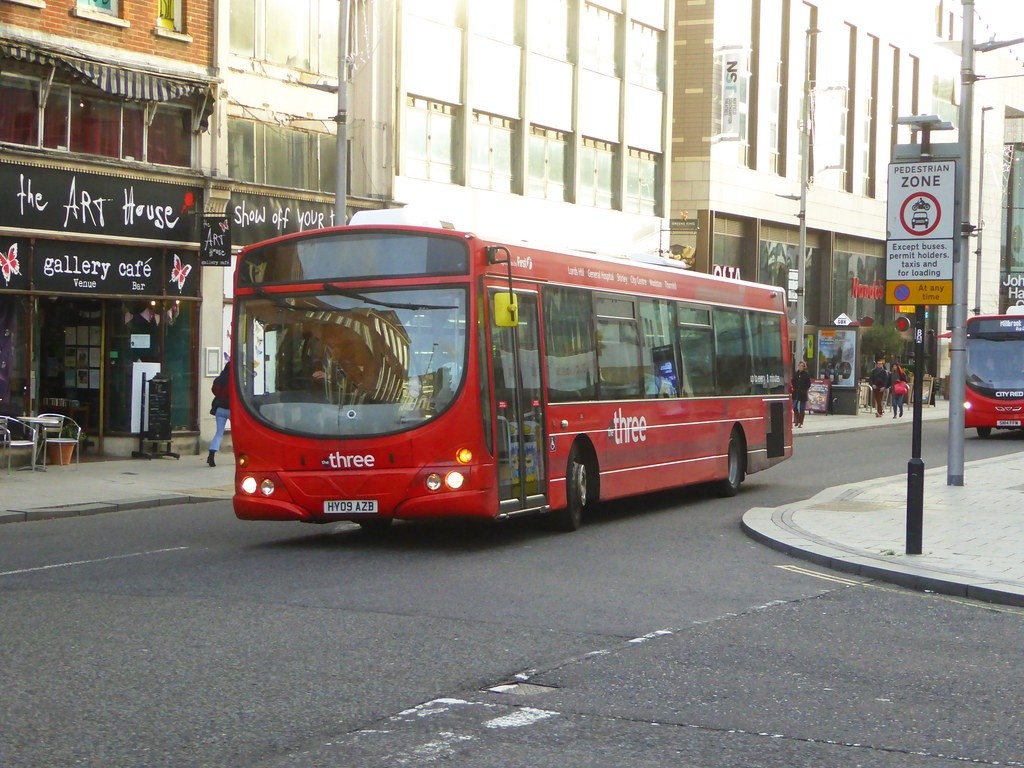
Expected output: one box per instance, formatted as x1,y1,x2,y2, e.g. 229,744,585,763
207,450,216,467
794,414,802,428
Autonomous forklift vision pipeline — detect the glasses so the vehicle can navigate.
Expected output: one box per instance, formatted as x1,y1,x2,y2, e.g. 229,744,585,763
798,364,803,366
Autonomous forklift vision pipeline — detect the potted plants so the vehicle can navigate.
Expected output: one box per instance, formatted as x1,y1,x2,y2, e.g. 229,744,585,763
38,427,86,465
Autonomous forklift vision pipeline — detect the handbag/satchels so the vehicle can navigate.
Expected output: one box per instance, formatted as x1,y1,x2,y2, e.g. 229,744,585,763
894,381,908,395
210,396,218,415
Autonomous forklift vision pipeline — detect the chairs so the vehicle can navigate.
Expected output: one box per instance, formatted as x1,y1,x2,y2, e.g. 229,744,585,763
0,416,36,473
32,413,81,471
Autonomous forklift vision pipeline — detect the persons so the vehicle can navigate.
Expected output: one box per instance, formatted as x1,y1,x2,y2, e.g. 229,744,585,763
791,361,812,429
869,361,890,417
981,359,1001,378
205,361,231,466
889,362,907,418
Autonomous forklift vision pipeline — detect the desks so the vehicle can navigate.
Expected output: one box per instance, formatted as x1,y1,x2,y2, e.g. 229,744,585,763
39,400,92,436
18,416,59,472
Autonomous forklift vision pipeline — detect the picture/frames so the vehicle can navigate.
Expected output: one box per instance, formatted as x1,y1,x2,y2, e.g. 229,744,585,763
205,347,222,377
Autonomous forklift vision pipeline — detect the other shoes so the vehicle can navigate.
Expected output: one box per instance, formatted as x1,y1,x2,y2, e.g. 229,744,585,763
875,412,881,417
893,416,897,419
899,413,903,417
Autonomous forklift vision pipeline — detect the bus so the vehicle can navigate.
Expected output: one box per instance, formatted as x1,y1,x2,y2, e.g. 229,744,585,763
965,314,1024,438
229,222,794,535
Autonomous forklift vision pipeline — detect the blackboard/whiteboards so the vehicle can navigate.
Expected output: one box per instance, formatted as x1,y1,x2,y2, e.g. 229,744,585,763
148,380,171,440
